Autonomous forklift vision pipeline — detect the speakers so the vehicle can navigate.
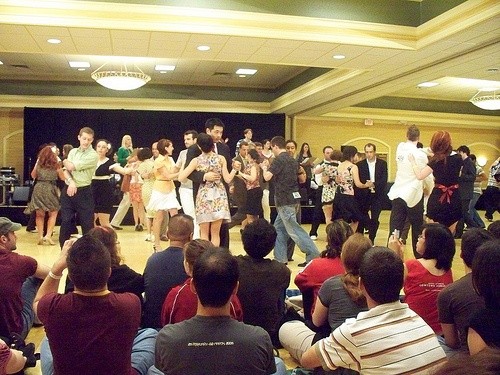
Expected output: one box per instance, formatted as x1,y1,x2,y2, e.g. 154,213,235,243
300,188,307,203
13,187,31,205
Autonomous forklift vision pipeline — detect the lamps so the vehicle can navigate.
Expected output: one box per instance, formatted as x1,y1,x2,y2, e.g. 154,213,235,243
469,85,500,111
90,57,152,92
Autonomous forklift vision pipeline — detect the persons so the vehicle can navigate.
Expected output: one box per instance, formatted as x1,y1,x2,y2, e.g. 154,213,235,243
354,143,388,245
389,222,455,337
467,239,500,355
438,228,495,351
452,146,481,238
484,156,500,221
279,219,446,375
487,220,500,240
409,130,464,234
24,117,278,252
34,234,158,375
0,217,52,375
141,213,294,375
464,154,488,231
257,136,320,267
285,140,340,261
332,146,374,233
388,128,435,257
63,226,146,309
399,142,423,245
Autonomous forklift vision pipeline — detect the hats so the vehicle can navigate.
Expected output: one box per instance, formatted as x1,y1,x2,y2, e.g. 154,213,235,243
0,217,23,236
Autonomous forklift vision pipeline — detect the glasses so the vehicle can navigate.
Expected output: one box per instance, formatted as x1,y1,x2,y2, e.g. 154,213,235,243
115,240,121,246
416,234,426,242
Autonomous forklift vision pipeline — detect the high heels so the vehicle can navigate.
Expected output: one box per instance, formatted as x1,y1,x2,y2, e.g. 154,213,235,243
39,237,43,245
45,237,56,245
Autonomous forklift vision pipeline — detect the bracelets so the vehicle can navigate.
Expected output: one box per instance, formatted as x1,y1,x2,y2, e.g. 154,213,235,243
49,271,63,279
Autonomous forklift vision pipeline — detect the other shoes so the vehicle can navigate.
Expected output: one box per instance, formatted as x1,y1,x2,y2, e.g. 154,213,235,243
32,322,44,327
311,235,317,240
400,238,406,245
153,246,163,252
110,224,123,230
287,258,294,261
10,333,26,351
160,235,168,241
453,235,461,238
298,262,308,267
486,216,492,221
146,234,152,240
135,224,144,230
20,342,36,368
27,227,36,231
